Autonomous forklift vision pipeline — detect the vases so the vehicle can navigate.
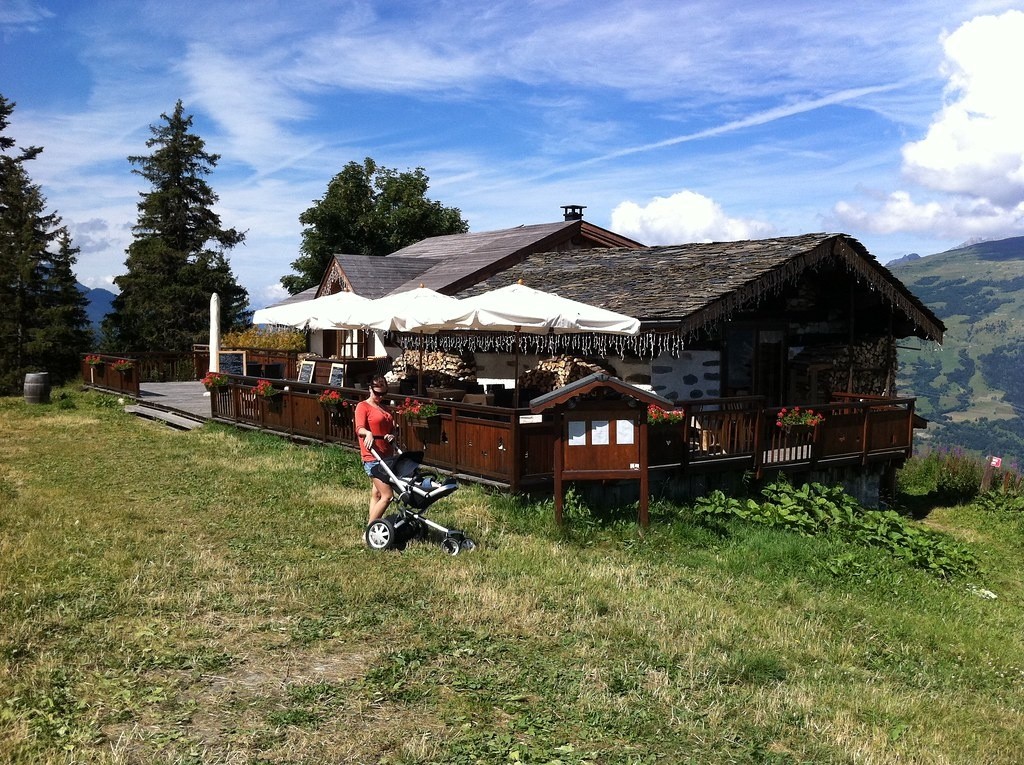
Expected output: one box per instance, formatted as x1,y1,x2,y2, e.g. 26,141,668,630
89,363,104,369
649,422,682,432
204,384,228,393
406,414,440,429
258,392,283,402
786,424,815,433
117,369,130,375
323,402,342,413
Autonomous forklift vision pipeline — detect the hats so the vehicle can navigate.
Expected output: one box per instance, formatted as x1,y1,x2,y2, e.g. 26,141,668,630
396,458,418,477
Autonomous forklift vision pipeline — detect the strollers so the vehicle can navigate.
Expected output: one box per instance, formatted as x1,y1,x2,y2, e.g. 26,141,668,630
365,435,477,556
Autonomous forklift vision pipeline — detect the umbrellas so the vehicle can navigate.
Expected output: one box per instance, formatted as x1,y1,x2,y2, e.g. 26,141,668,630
253,279,640,409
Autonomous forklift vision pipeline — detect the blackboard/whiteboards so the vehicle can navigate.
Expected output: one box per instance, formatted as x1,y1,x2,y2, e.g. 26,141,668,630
296,361,316,383
215,350,247,375
327,363,347,387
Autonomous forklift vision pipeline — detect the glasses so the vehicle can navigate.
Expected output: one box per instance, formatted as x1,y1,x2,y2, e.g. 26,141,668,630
372,387,387,396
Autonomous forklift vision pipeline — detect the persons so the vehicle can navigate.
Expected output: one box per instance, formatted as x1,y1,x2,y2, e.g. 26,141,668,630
354,374,397,541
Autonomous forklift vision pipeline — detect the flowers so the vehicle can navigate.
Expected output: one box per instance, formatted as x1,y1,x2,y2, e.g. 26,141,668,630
776,406,826,427
316,389,348,407
250,379,278,397
395,398,438,418
110,359,135,371
201,369,230,388
646,404,684,426
81,355,104,370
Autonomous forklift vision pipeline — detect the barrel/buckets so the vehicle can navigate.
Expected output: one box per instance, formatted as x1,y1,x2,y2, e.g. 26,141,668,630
24,373,51,404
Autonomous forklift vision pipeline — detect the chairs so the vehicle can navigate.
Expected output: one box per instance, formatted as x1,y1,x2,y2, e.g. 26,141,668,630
466,385,485,394
399,379,428,398
246,364,263,377
487,384,510,407
264,362,285,379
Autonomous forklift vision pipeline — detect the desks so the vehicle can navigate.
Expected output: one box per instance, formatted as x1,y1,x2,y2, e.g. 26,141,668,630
462,394,494,406
388,384,465,399
